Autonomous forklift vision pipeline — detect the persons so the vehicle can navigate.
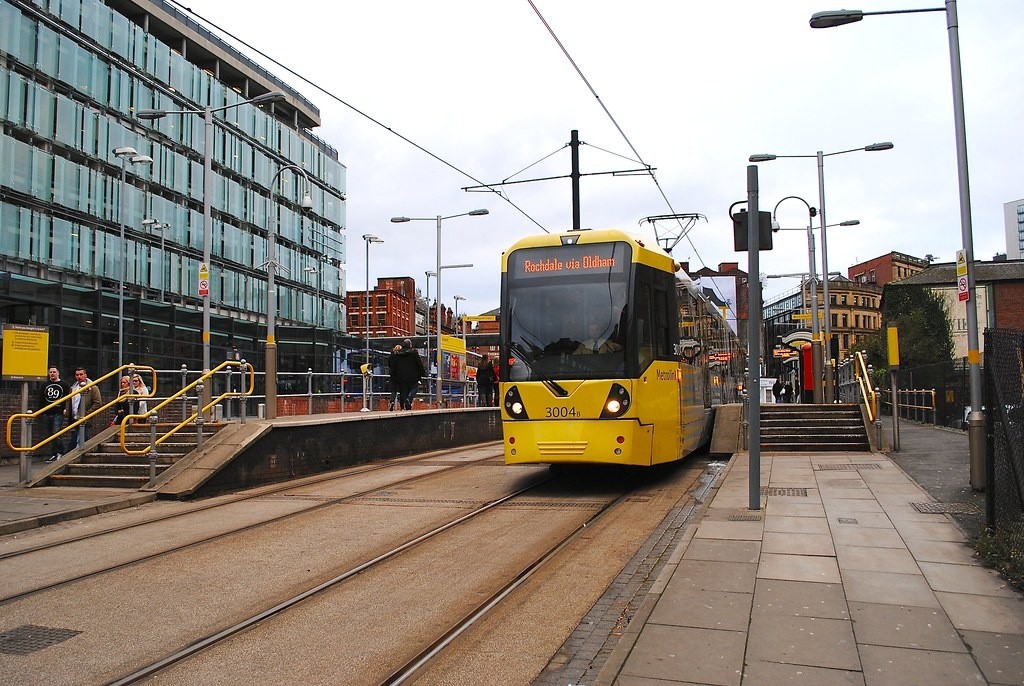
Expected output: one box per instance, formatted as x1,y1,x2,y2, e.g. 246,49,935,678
476,354,499,407
67,366,102,452
40,365,70,462
772,377,793,403
115,372,148,425
572,322,622,354
388,339,426,411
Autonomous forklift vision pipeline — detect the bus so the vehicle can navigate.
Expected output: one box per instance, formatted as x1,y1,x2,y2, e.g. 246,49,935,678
493,212,746,469
345,349,483,401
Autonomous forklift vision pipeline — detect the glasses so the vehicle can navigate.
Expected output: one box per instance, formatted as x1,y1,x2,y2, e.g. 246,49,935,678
132,380,140,383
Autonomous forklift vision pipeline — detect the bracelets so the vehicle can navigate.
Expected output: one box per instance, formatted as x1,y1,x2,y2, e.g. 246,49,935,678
122,409,124,412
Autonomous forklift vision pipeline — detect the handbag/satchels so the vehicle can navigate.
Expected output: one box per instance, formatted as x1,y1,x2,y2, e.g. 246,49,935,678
780,388,785,395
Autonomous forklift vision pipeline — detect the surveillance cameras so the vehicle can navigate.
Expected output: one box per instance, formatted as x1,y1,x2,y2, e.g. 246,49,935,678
301,196,313,213
771,221,780,233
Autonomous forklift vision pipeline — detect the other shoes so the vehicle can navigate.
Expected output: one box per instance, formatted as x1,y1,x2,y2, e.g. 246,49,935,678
389,402,395,411
401,406,406,411
404,396,412,410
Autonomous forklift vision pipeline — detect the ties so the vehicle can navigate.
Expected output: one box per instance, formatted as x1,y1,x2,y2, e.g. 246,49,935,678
593,341,599,354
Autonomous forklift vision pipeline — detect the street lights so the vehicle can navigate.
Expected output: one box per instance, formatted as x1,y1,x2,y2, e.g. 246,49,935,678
766,270,842,329
773,218,860,338
109,146,155,387
361,232,385,363
452,295,468,338
771,304,824,330
252,164,315,420
390,207,490,409
748,142,897,404
809,0,987,492
143,215,172,303
423,270,438,377
136,90,287,423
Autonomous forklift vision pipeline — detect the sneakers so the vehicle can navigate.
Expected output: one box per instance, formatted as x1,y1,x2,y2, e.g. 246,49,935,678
45,455,58,463
